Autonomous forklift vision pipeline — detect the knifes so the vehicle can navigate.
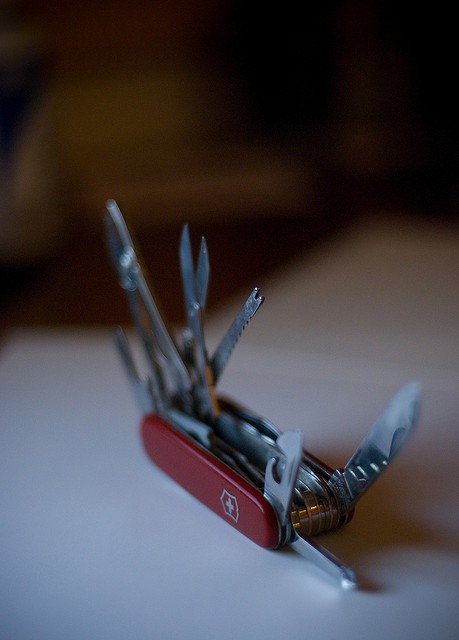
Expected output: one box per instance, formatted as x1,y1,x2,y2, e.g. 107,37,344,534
329,379,422,513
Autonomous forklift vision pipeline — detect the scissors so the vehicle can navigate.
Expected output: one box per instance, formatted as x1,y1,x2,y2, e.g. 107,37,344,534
179,225,220,424
103,201,190,405
